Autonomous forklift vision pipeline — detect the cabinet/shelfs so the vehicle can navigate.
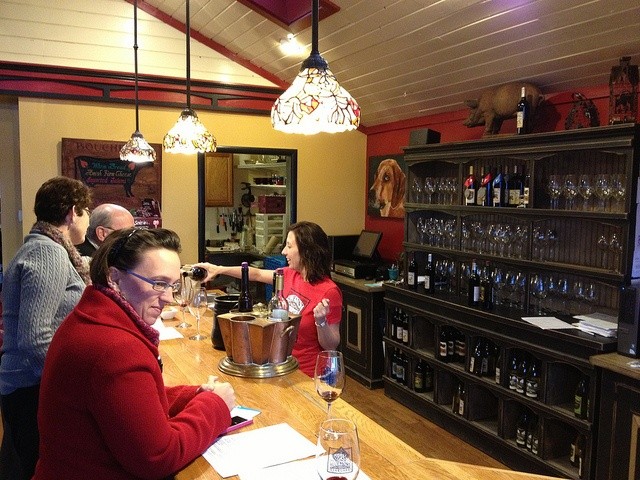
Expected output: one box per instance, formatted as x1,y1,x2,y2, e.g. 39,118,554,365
589,352,640,480
237,154,290,196
255,212,287,249
399,123,639,326
330,273,396,391
382,275,618,480
206,154,233,206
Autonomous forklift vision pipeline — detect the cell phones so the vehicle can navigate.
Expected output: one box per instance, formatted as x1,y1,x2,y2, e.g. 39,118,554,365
218,416,252,437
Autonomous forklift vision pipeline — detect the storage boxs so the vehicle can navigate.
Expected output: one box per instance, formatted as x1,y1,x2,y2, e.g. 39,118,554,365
258,195,286,214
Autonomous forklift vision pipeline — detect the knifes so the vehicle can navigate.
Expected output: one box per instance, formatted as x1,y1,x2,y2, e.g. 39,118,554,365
216,207,220,233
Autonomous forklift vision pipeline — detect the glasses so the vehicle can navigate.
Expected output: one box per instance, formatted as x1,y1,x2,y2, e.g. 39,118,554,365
83,207,93,216
127,271,182,294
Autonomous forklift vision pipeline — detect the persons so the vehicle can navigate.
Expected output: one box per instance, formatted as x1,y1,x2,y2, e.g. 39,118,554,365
31,227,236,480
0,177,94,472
190,221,342,381
74,204,135,274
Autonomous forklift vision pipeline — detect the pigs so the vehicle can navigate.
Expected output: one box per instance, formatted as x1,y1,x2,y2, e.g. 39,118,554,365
463,82,545,135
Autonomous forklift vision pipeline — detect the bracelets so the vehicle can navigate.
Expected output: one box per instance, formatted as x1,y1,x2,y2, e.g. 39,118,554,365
315,318,327,328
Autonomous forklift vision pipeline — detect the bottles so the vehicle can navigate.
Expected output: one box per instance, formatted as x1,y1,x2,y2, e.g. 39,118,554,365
438,331,465,361
568,432,585,478
424,254,435,294
467,259,481,306
414,359,432,393
452,381,464,416
238,262,254,311
268,270,289,323
391,345,408,386
516,410,540,455
482,260,497,312
517,85,530,133
469,341,500,384
391,307,409,345
509,357,541,398
407,253,417,290
182,267,207,282
574,377,592,421
465,164,528,205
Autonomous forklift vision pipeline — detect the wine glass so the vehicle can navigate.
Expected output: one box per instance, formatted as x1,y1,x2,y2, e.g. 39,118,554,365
531,224,558,262
460,261,471,296
434,259,456,294
174,280,195,328
547,172,627,211
529,269,599,319
315,350,346,440
493,266,527,310
461,216,528,259
608,231,624,270
597,228,610,267
413,176,457,205
187,288,207,342
416,215,456,250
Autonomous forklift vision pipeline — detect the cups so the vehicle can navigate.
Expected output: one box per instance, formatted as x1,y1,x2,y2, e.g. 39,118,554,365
316,420,360,480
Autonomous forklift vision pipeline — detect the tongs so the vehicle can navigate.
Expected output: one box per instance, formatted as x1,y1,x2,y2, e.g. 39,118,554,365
231,208,237,236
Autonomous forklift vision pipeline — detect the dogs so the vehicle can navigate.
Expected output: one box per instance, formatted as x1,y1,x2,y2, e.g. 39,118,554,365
369,158,405,218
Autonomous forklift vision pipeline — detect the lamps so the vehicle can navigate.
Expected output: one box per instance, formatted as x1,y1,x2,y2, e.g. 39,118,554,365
270,1,362,134
120,0,157,165
163,0,217,156
